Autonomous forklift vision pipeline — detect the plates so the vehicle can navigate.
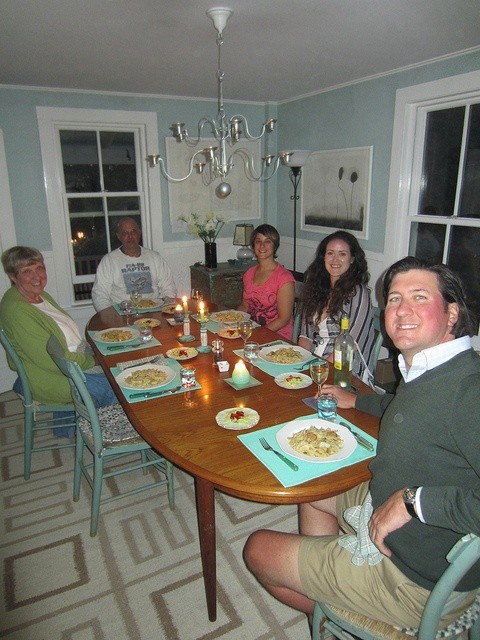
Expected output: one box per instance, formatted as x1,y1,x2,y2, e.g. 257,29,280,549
115,365,176,391
94,327,140,345
215,407,259,430
275,372,312,389
258,344,311,365
131,298,164,309
224,375,264,392
219,328,240,339
209,310,250,324
161,305,179,312
276,419,358,464
134,318,160,328
166,317,185,327
165,347,198,361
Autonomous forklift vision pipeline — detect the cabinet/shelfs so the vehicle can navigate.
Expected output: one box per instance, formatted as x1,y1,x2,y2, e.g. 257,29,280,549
190,259,258,308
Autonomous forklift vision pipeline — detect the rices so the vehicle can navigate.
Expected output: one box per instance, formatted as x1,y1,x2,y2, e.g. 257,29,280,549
266,347,302,364
289,426,342,457
214,311,246,321
139,299,155,308
124,367,164,389
102,329,132,342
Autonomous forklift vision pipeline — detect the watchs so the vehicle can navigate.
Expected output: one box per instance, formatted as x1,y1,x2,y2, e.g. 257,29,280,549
403,485,420,520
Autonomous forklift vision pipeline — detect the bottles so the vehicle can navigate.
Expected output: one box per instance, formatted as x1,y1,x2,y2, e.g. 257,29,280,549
211,340,224,367
333,316,354,392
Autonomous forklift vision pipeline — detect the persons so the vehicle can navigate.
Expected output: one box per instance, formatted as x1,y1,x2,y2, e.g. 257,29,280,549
242,257,480,640
298,231,375,386
234,224,296,340
0,245,119,407
91,217,178,313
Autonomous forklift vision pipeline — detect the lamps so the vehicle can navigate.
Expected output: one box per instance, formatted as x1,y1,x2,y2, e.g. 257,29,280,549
233,223,255,265
145,8,291,201
278,150,314,280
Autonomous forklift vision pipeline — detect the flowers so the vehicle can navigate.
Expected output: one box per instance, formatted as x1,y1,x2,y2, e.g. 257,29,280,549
177,211,228,243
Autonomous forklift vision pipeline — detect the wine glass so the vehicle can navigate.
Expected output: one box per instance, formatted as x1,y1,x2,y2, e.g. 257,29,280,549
191,288,203,315
310,360,329,398
237,321,253,350
131,291,142,316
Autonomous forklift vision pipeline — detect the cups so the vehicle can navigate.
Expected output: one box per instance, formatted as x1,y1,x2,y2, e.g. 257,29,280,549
243,342,259,363
140,326,151,343
121,301,132,314
318,395,337,421
180,368,196,389
196,307,208,323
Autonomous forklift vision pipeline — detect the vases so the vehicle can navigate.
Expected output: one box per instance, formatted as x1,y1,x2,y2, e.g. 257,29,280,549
205,242,217,268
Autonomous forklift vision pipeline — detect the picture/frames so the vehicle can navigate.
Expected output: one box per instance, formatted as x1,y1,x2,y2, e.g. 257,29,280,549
163,136,264,227
298,145,374,242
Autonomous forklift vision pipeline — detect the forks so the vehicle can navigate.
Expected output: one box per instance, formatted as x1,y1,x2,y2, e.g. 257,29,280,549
258,437,298,470
293,358,319,370
122,355,161,370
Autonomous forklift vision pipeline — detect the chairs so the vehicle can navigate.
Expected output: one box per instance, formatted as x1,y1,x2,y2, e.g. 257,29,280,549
290,279,307,345
369,303,387,373
312,533,479,640
68,361,176,538
0,328,88,483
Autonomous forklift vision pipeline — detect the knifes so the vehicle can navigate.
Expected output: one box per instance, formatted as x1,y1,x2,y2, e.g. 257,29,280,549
340,421,373,445
145,386,182,398
